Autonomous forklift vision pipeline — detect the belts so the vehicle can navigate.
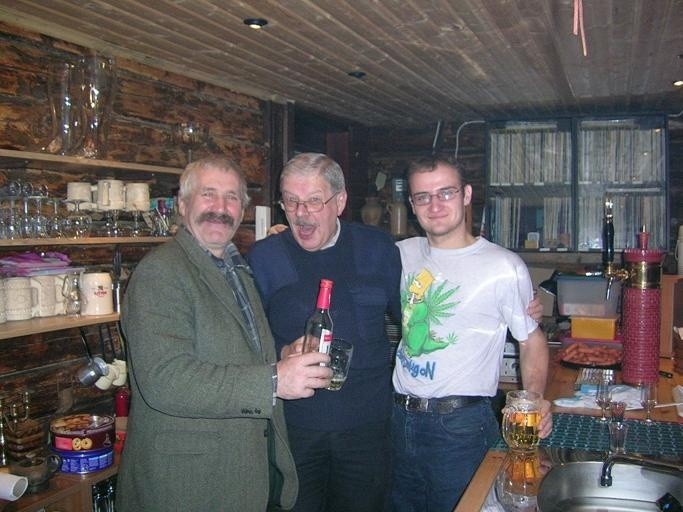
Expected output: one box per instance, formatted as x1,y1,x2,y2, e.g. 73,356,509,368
395,392,483,415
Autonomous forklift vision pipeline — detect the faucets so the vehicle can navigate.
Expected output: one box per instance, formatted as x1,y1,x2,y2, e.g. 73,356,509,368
600,196,625,300
601,419,682,486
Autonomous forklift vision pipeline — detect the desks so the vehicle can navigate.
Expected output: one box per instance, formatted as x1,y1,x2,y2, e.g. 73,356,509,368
453,346,683,512
0,421,122,512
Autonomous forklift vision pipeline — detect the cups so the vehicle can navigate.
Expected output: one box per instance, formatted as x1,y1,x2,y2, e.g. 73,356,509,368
66,179,148,211
608,401,628,455
502,391,542,454
0,272,114,324
325,337,354,392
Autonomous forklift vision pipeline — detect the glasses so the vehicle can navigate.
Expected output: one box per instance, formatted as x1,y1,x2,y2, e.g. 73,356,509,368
277,192,338,213
410,185,464,207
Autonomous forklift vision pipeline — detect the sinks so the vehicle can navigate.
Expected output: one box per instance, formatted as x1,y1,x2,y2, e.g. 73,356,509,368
536,460,683,512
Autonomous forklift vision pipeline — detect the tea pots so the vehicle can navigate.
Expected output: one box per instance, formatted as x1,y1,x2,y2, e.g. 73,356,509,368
5,451,63,495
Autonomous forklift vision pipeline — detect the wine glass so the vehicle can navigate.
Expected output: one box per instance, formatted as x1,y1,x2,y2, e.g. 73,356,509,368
642,378,657,426
597,379,611,422
174,121,210,163
0,194,91,239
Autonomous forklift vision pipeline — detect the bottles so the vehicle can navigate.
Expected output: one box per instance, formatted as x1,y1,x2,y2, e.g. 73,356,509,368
302,279,334,368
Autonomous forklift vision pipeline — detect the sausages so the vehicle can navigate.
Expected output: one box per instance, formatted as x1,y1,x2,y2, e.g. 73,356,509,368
562,343,620,366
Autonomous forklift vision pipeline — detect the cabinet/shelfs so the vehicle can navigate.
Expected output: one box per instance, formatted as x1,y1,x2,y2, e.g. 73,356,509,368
485,115,672,256
1,147,263,342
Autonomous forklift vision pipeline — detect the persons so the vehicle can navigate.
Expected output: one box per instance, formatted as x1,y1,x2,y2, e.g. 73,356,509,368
119,154,334,511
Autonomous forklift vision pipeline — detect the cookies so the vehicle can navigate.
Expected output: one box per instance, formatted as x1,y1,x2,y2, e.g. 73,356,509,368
72,438,81,451
81,438,93,450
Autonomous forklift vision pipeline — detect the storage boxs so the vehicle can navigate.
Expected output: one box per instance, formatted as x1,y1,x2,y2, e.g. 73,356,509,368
557,269,622,343
